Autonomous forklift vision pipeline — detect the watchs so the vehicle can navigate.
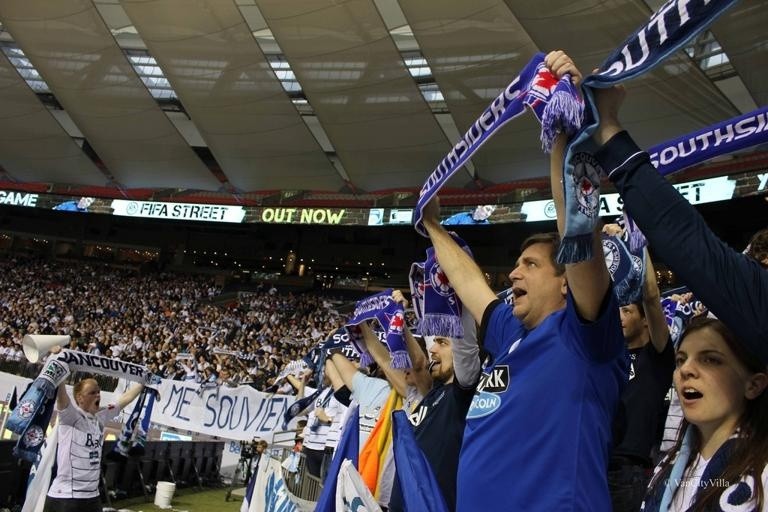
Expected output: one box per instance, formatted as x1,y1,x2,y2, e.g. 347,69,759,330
327,416,333,425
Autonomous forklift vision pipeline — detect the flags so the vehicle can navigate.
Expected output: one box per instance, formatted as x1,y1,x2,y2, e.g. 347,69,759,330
9,386,18,411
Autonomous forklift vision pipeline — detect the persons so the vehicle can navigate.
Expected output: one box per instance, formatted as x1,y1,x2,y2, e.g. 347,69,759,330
656,287,708,344
45,345,152,511
751,231,768,266
420,50,627,512
256,440,268,455
0,252,419,396
590,74,767,374
269,231,480,512
439,205,498,225
51,196,95,212
602,215,674,512
639,317,768,512
